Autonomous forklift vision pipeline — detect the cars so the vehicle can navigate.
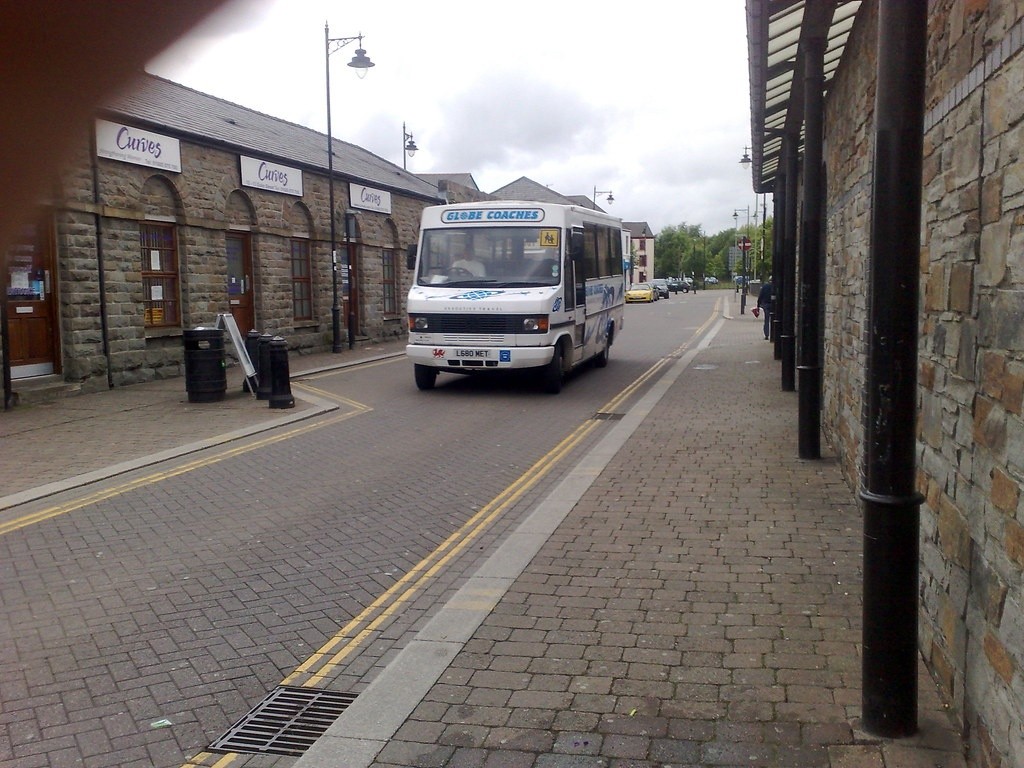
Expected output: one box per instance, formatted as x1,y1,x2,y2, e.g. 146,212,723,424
648,282,661,304
650,276,693,299
625,282,656,303
704,277,719,284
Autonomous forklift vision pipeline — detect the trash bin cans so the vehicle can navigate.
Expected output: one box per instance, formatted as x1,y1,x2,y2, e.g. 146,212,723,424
184,326,227,404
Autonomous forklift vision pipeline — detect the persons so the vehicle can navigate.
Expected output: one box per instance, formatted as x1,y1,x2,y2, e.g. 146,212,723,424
451,244,487,280
757,275,772,340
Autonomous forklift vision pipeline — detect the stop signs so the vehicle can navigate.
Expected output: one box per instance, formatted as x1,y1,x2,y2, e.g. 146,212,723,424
737,238,752,251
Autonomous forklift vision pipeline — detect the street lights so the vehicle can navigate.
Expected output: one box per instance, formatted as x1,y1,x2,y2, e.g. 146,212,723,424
753,206,767,283
402,121,418,170
323,20,374,353
593,185,614,210
732,205,749,294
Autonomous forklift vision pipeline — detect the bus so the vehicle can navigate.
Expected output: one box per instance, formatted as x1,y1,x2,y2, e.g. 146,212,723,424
404,199,629,395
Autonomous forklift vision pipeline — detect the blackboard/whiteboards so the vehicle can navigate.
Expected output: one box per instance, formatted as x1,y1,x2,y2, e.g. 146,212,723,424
222,313,257,379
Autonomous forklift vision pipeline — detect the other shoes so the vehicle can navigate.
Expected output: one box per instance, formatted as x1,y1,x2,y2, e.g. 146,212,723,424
765,336,769,339
